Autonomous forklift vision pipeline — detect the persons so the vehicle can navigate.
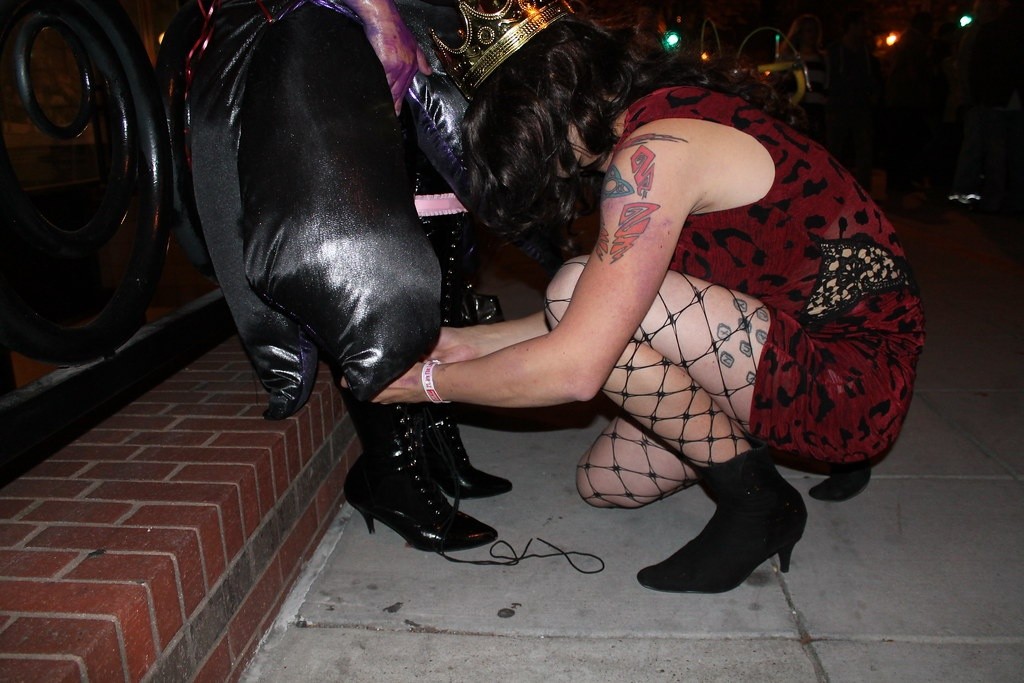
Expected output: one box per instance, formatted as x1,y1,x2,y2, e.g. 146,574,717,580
831,9,882,166
779,15,827,142
341,0,925,593
156,0,513,551
884,11,932,192
931,0,1024,215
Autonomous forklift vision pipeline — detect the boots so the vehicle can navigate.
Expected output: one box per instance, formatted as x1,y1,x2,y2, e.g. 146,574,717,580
330,359,498,553
809,464,872,500
405,357,513,498
638,436,808,594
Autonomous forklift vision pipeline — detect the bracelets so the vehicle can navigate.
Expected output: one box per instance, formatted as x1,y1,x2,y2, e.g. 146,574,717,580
422,360,451,403
